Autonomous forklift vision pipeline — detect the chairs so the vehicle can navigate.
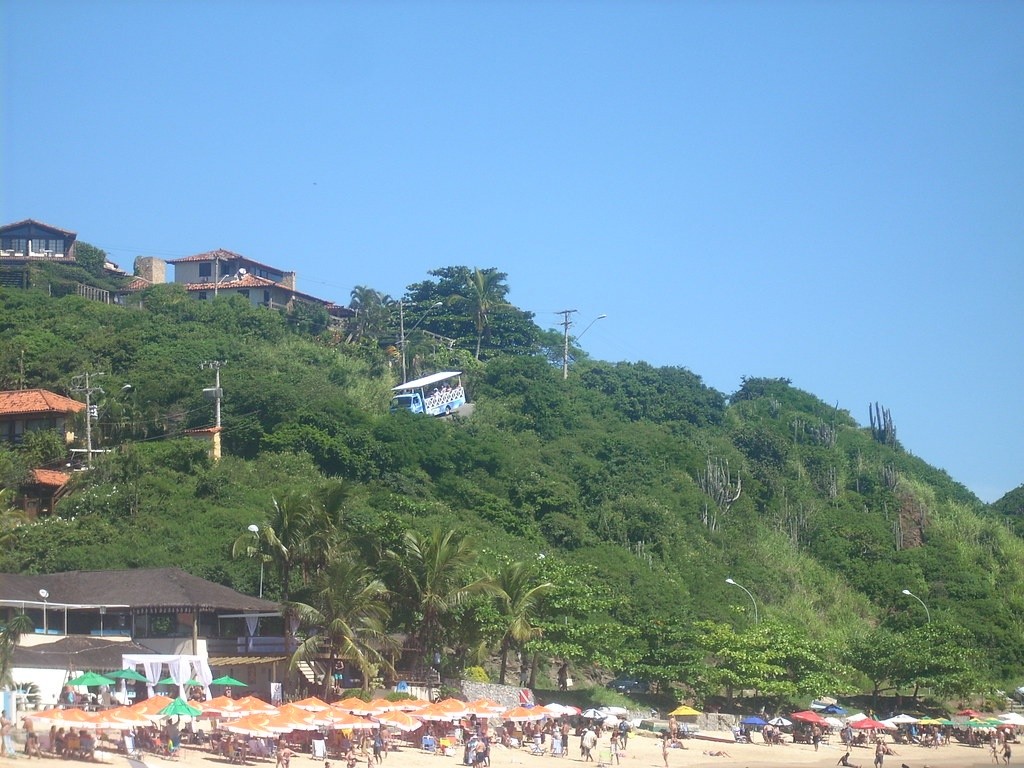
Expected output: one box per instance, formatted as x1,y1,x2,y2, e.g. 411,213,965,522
311,739,328,761
911,737,929,746
598,749,611,766
207,732,272,765
123,736,146,761
2,734,18,759
529,745,548,756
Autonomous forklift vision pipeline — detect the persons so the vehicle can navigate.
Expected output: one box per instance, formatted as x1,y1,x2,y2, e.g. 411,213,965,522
661,717,687,768
0,709,633,768
426,380,452,398
744,724,1021,768
704,750,731,757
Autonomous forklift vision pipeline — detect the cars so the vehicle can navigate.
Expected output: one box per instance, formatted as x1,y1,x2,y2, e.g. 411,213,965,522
605,673,652,696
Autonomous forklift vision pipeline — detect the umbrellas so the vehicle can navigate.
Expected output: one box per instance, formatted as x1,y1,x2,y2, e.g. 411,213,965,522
31,693,629,761
67,667,248,697
741,702,1024,733
668,705,701,715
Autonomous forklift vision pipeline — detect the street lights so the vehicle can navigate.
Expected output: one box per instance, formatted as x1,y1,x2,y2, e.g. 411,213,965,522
397,299,444,382
726,577,758,631
249,524,267,598
85,383,132,469
562,315,606,381
214,273,229,299
902,589,931,623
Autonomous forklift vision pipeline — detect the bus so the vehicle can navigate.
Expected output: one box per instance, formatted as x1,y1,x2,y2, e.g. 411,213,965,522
389,372,466,421
